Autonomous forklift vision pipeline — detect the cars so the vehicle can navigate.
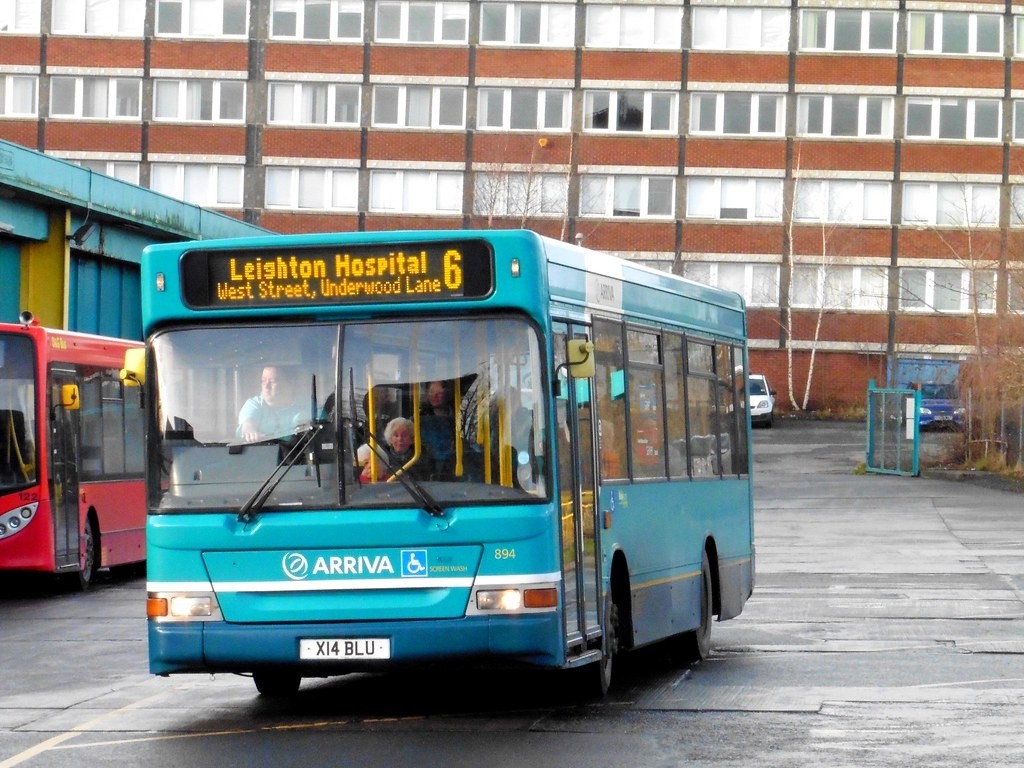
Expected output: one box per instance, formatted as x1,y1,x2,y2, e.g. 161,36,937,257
901,380,968,433
748,372,776,431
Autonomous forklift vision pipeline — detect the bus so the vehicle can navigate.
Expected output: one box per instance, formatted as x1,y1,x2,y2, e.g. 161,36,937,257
116,229,756,706
0,312,243,596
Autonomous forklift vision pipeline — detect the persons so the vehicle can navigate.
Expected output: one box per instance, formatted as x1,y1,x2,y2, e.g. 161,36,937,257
235,362,330,442
357,378,536,484
